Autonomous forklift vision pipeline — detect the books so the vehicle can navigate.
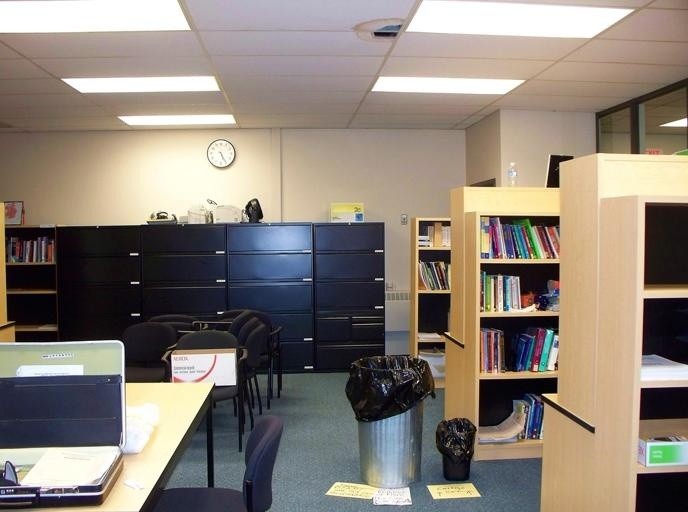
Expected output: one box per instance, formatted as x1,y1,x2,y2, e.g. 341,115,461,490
641,353,688,380
418,332,441,342
417,259,450,291
547,335,560,370
479,216,560,259
419,222,451,247
541,289,560,312
480,327,505,372
522,334,536,371
39,223,55,227
528,326,546,372
539,328,553,372
5,237,54,263
512,338,527,372
478,394,545,444
479,269,521,312
418,348,445,378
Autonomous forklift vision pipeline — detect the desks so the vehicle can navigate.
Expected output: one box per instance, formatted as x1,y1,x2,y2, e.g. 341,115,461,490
1,381,216,512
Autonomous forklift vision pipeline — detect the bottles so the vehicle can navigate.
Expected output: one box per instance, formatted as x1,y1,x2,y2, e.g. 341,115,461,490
507,162,517,187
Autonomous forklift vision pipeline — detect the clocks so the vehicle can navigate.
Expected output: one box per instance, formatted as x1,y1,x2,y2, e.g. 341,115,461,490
206,138,236,168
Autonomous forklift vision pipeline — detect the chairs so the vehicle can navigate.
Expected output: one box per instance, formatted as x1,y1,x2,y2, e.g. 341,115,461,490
149,314,196,326
122,323,176,382
161,330,249,452
229,309,283,430
153,415,284,512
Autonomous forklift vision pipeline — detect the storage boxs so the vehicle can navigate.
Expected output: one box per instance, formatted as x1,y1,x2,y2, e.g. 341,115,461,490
171,348,238,387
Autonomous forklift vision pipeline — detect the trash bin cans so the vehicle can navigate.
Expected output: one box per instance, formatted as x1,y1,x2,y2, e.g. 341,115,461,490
437,418,477,480
352,356,431,489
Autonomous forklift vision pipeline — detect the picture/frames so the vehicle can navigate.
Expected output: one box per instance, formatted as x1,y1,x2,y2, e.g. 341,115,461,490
4,200,24,226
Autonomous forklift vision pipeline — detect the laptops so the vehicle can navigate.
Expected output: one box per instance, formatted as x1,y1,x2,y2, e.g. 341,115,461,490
0,340,127,443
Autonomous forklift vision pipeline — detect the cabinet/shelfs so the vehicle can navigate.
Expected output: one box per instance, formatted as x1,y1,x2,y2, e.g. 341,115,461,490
227,222,314,374
141,222,228,318
600,194,687,510
3,225,58,341
56,225,144,343
464,210,561,462
313,221,386,373
412,216,451,390
